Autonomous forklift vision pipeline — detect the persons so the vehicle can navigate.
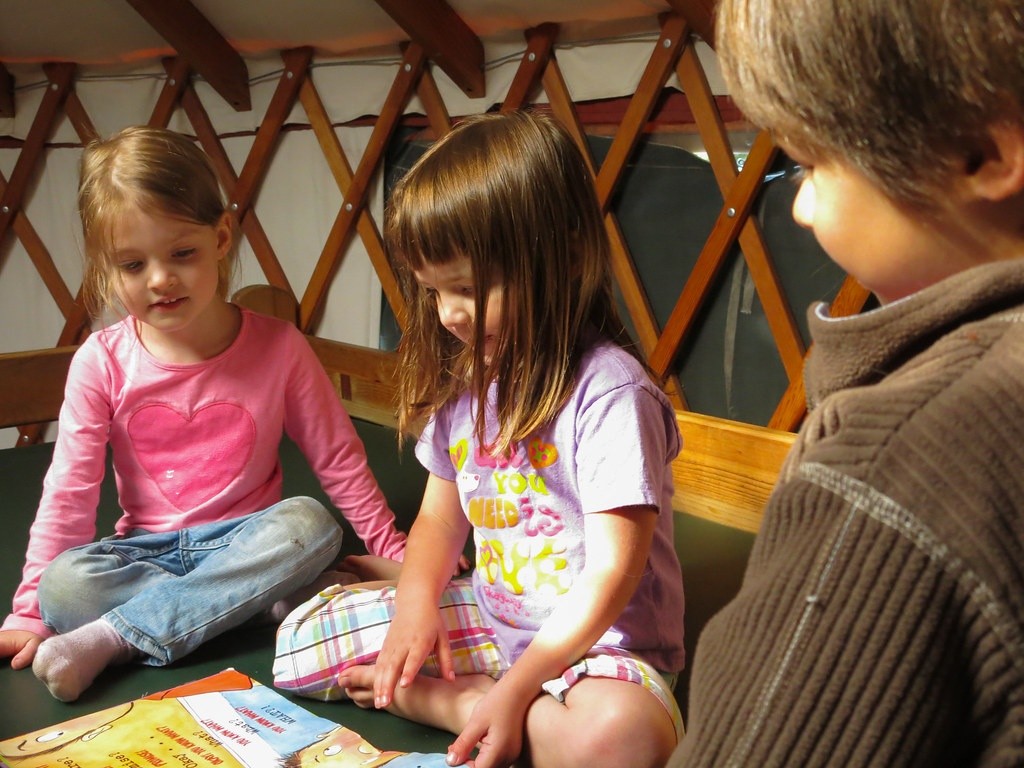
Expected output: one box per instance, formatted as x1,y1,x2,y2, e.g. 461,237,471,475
270,108,688,768
0,126,469,702
662,0,1024,768
